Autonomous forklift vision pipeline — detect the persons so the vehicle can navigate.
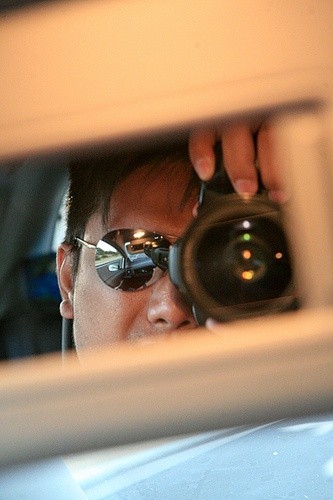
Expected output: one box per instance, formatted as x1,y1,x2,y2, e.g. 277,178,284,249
53,123,299,360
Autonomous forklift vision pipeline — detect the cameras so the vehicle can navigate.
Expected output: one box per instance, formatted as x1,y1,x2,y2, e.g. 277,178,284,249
151,165,302,324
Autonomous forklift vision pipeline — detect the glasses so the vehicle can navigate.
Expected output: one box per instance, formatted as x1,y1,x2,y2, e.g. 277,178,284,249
73,228,171,292
